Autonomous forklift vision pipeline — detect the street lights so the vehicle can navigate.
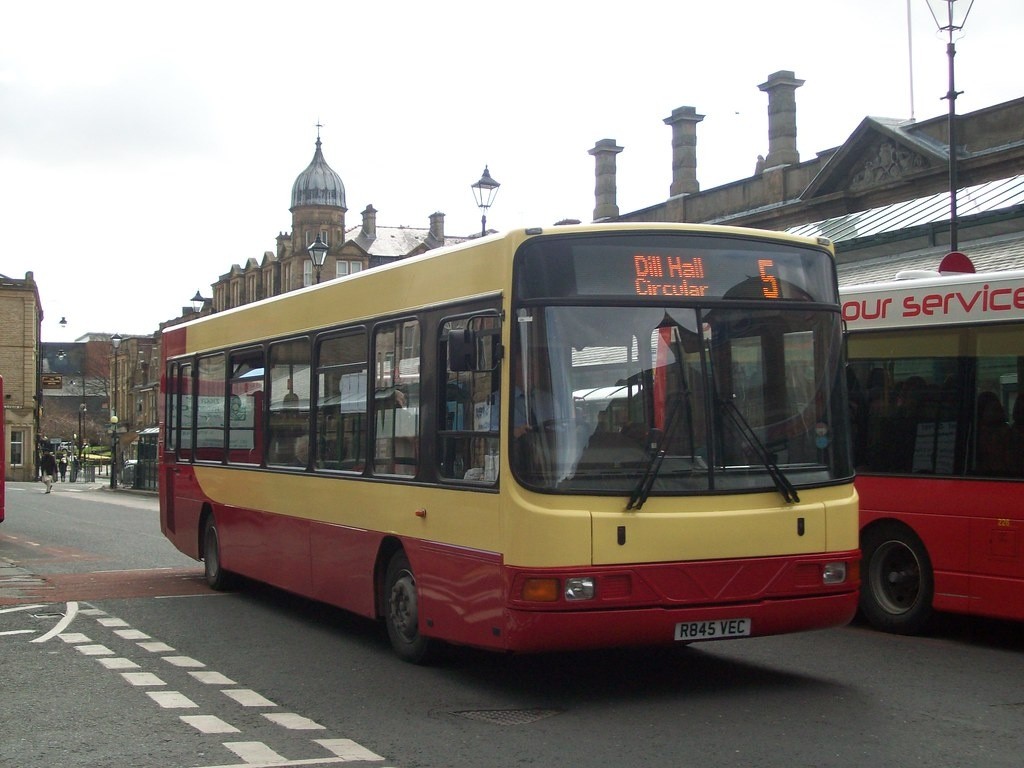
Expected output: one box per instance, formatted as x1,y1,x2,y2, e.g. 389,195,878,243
77,403,87,457
68,375,87,440
926,0,975,256
109,416,120,489
470,163,502,238
109,333,122,421
306,235,329,282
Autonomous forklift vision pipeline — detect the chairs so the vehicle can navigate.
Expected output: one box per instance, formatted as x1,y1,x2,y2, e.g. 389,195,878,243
176,447,260,465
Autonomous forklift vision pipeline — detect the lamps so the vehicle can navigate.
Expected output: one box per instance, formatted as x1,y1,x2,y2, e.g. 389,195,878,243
59,317,67,324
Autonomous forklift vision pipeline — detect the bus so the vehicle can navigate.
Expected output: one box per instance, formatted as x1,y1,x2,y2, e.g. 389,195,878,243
670,271,1024,631
157,223,861,658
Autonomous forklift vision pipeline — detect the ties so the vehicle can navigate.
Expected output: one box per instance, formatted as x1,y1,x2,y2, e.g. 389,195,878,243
527,396,537,432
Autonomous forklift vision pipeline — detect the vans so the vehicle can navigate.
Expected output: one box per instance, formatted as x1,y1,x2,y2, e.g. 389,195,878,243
58,441,89,452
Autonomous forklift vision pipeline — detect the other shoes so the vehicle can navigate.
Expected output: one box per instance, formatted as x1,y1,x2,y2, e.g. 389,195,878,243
45,491,50,494
50,486,52,490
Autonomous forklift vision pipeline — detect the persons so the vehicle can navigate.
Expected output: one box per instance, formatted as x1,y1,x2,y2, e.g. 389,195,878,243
482,352,561,439
40,448,57,493
58,457,67,482
291,434,308,467
74,456,79,477
